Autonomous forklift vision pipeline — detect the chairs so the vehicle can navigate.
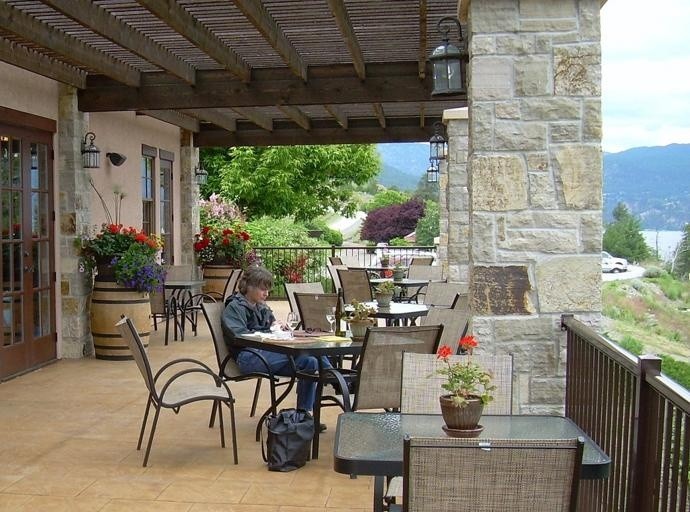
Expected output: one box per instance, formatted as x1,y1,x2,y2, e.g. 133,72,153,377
181,269,242,341
145,278,177,345
114,313,238,467
201,304,295,419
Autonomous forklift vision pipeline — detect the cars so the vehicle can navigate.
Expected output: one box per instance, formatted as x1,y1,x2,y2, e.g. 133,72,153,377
601,250,628,274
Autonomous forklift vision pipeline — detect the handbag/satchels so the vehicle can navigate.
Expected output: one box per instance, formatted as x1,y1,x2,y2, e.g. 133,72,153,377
267,408,315,472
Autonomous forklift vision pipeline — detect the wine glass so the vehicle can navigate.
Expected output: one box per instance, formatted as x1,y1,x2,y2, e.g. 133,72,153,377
286,312,298,340
327,307,336,333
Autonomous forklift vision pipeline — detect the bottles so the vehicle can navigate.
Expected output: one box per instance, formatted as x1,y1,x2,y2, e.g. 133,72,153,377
335,288,348,337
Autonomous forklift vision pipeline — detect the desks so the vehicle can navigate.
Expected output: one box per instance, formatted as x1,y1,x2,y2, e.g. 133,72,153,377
238,328,422,458
161,280,209,341
334,411,613,511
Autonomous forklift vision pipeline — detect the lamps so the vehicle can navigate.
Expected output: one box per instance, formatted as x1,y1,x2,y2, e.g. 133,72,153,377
195,161,208,185
428,15,468,96
427,158,439,182
81,132,101,169
429,121,447,160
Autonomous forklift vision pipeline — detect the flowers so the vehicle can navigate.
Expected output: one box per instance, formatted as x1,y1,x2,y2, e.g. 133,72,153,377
239,247,261,269
195,226,250,267
108,253,167,294
74,223,163,285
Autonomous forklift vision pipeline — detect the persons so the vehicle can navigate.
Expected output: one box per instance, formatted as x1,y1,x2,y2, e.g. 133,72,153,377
218,265,354,433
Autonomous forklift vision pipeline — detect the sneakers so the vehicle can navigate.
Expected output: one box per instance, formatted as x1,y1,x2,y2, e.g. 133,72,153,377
304,412,327,432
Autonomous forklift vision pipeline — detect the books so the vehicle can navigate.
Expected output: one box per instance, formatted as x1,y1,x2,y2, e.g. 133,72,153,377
283,328,333,338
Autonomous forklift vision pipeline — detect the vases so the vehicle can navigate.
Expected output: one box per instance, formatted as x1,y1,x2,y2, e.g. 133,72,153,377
213,249,233,264
95,255,121,281
